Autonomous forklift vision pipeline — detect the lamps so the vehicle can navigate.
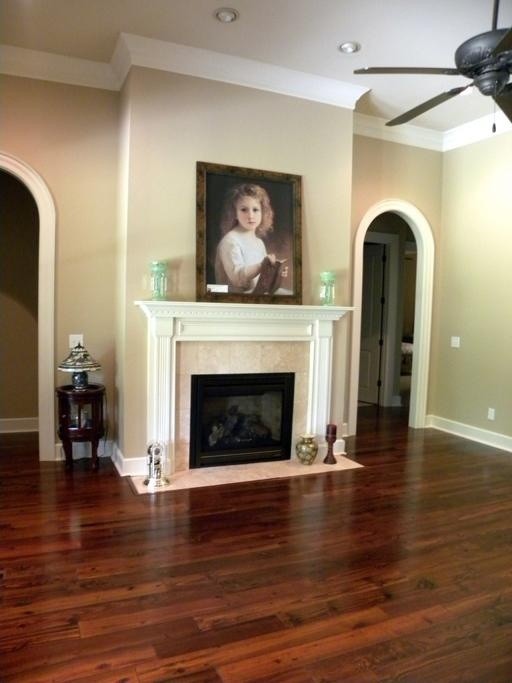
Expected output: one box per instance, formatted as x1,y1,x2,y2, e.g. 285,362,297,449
58,344,101,390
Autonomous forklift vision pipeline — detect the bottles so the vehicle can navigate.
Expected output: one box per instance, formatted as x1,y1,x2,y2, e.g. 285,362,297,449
151,259,170,301
320,271,337,306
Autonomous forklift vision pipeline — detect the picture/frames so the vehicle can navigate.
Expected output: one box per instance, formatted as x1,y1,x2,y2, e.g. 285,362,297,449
194,159,303,305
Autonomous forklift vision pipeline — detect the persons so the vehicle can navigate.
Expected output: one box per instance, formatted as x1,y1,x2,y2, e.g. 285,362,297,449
215,183,293,295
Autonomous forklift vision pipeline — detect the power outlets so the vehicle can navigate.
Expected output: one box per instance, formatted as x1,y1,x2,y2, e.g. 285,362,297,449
488,408,495,421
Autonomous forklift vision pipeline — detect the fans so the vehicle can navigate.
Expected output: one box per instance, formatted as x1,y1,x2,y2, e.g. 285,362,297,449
354,2,512,133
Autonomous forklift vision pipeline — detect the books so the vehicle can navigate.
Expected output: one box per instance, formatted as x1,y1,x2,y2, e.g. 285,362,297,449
253,258,288,294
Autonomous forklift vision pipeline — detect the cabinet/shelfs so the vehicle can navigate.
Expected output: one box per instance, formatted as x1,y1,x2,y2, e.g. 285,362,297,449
56,384,104,468
